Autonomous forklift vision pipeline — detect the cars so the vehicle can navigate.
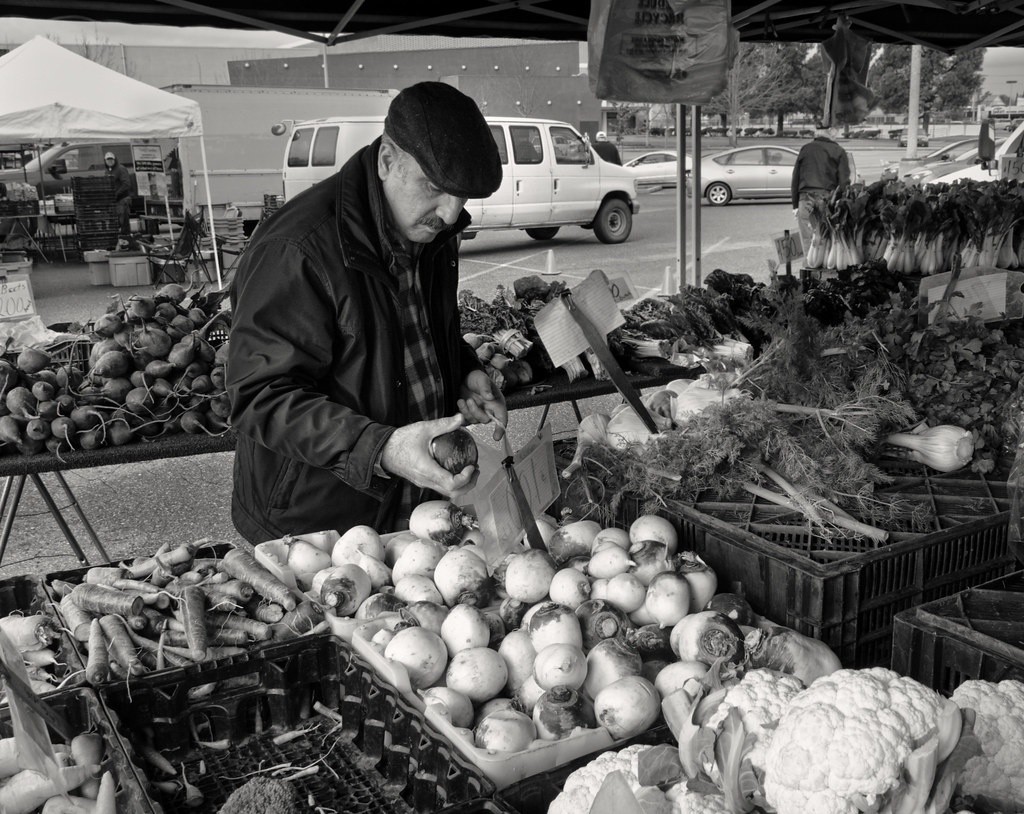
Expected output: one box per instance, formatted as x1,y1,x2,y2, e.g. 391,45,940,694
881,135,1010,188
903,138,1011,189
900,128,930,147
622,150,697,189
687,145,865,206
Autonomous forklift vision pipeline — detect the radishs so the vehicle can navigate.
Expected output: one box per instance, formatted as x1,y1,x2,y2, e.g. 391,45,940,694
288,500,842,753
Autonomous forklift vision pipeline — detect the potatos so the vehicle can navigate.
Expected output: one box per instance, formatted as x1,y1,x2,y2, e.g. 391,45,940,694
0,286,231,453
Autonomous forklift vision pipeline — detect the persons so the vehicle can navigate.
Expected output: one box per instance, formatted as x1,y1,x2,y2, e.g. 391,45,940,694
791,120,851,269
104,152,132,235
591,131,622,166
223,81,508,546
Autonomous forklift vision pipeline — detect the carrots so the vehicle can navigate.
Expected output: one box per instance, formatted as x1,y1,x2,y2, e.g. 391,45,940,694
0,539,324,814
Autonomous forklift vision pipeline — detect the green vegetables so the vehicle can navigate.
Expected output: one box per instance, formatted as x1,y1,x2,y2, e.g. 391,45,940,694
458,178,1024,532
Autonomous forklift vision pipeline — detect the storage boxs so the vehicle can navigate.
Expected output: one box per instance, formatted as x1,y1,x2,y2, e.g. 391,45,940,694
0,176,286,286
0,434,1024,814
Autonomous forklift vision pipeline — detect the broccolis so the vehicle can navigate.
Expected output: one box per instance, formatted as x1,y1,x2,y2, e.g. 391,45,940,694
547,659,1024,814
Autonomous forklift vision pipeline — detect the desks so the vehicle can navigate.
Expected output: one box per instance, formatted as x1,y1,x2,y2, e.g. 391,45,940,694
147,200,183,215
44,211,75,264
0,361,698,573
0,213,49,264
140,215,184,234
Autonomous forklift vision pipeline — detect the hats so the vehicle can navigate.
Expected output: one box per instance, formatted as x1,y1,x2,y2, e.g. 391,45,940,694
385,81,502,200
105,152,115,160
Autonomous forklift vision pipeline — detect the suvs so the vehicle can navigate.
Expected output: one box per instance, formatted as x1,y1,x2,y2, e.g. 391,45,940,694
1,136,157,204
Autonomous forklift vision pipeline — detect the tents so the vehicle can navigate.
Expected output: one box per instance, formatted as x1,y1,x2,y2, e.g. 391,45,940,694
0,36,222,291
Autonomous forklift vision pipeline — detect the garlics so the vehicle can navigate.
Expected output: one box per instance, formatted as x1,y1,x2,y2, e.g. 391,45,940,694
888,425,973,472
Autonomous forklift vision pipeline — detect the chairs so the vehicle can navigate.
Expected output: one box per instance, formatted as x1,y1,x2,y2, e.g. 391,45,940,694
147,207,213,290
771,153,783,165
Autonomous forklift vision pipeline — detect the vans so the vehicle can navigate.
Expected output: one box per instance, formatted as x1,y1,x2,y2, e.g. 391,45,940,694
281,115,640,245
921,116,1024,195
